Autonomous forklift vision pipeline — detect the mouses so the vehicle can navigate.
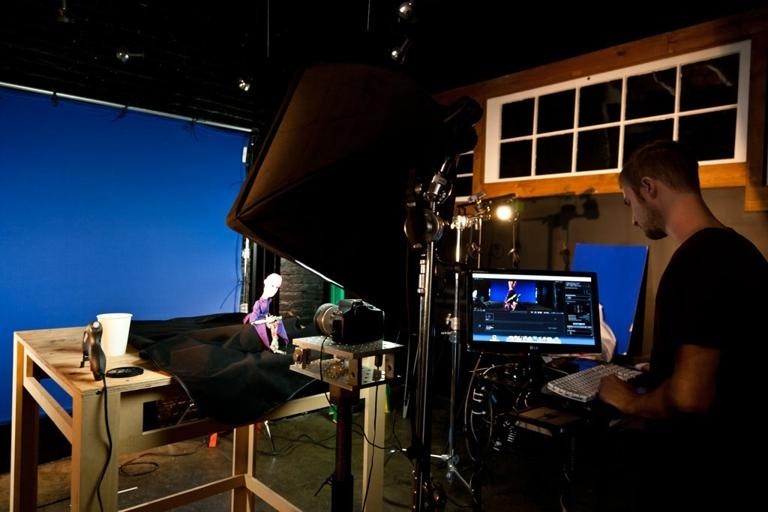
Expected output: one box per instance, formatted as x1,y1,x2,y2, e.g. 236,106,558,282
634,361,650,372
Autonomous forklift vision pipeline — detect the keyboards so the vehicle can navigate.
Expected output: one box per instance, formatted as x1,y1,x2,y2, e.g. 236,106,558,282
542,363,644,404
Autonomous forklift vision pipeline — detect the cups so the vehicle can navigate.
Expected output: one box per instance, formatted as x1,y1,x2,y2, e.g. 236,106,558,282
95,312,134,357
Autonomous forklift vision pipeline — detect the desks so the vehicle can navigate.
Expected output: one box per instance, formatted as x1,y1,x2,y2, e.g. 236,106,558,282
9,327,406,511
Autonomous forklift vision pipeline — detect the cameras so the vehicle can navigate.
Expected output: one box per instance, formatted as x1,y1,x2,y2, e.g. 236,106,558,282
314,298,384,345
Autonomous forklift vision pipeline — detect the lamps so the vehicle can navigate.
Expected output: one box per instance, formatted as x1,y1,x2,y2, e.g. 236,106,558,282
227,61,486,511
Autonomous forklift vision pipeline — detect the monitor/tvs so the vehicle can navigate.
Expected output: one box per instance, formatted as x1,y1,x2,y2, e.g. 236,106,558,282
464,266,602,386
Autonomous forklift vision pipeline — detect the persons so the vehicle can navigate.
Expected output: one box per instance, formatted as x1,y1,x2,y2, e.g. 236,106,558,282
599,136,762,510
244,272,289,357
